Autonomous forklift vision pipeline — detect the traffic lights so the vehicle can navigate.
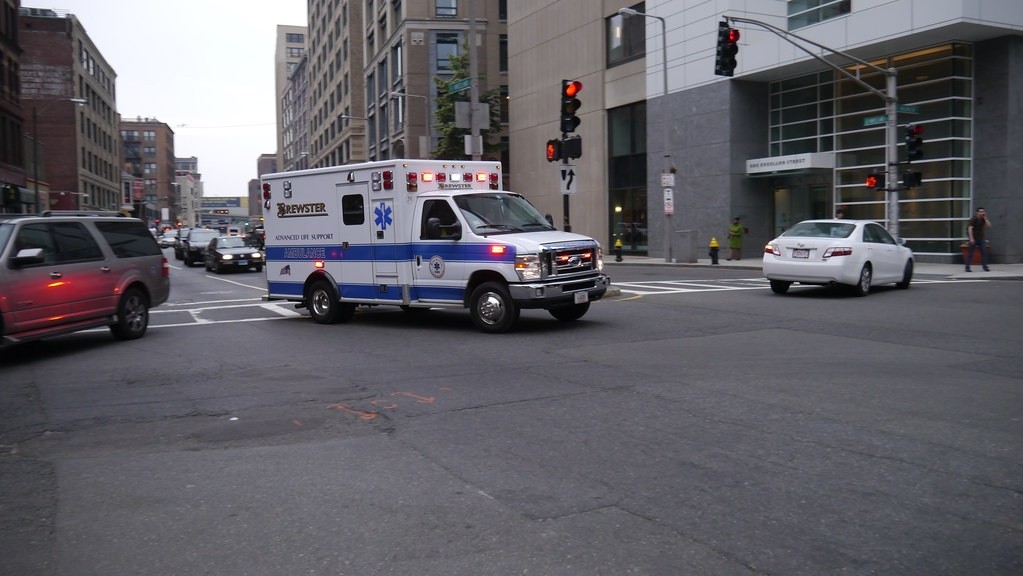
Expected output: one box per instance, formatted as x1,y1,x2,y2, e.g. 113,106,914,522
714,21,740,77
546,79,582,162
905,124,923,159
866,173,880,188
216,209,228,214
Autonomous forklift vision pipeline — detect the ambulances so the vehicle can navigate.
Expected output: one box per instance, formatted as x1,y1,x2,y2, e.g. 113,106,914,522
260,160,611,333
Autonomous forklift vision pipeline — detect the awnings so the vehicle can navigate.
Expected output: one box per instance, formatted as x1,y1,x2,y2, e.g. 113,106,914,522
3,186,36,204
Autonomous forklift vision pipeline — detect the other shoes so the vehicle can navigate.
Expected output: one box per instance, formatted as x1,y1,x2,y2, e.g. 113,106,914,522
736,257,740,260
966,269,972,272
985,268,990,271
726,257,732,260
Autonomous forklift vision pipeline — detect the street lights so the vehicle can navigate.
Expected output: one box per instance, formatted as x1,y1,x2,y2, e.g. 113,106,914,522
300,152,320,167
341,115,372,161
32,98,88,213
618,8,668,153
139,181,180,219
391,92,431,159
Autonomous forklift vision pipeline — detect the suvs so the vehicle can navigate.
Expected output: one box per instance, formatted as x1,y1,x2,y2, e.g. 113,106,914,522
182,227,222,267
174,228,189,261
0,210,169,345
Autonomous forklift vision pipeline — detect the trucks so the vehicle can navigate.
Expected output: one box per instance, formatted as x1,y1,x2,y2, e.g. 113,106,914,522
226,226,241,236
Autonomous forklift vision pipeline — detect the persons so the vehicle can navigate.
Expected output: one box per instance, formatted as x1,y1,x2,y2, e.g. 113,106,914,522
831,209,848,235
726,218,743,261
966,208,992,272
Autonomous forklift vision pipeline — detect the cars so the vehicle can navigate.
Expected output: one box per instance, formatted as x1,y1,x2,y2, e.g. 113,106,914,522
763,218,914,296
148,228,178,249
204,237,262,273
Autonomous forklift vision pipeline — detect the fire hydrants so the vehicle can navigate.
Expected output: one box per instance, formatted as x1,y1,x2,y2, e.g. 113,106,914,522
614,239,623,262
708,237,719,264
